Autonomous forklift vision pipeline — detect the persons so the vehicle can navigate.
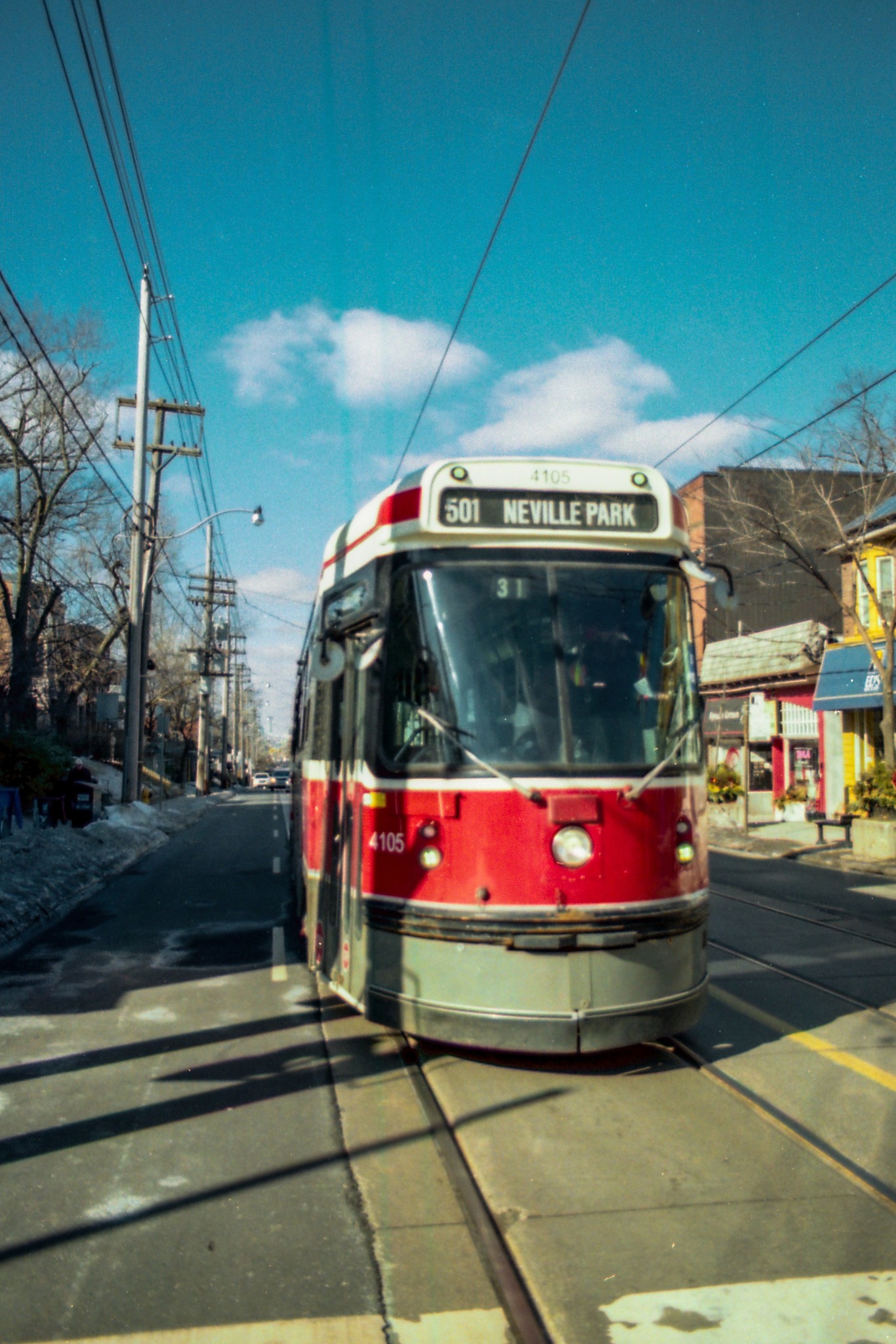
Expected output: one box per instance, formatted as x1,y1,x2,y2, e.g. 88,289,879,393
69,759,91,782
580,614,641,762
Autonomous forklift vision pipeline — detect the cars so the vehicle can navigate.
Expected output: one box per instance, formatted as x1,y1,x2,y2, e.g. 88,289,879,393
252,758,293,792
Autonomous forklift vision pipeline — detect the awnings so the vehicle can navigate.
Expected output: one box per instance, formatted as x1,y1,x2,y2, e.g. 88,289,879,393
813,639,896,711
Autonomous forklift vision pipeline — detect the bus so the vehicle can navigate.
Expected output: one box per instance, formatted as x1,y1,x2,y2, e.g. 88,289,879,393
284,457,743,1052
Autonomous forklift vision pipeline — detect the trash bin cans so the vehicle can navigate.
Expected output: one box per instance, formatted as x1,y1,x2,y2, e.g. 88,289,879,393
37,795,68,829
72,782,102,829
0,787,23,839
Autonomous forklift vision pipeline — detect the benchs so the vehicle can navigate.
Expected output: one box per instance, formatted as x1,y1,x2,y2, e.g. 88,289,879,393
814,820,852,849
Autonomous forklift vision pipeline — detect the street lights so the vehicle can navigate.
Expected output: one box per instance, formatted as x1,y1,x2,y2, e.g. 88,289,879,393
120,506,266,804
